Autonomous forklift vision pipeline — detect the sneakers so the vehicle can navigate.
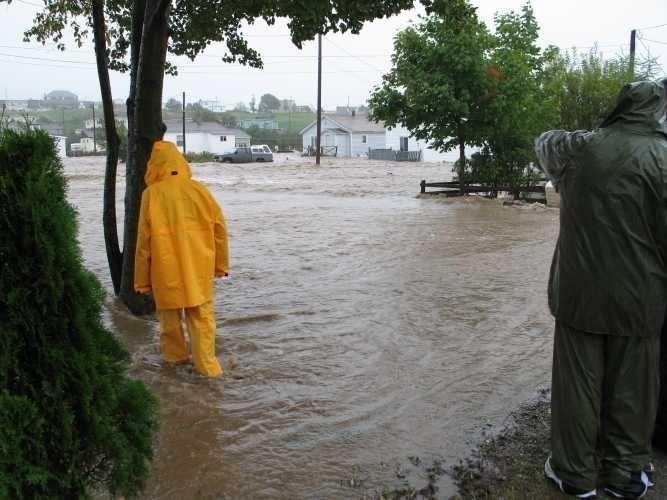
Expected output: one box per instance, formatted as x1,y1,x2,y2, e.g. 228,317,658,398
544,456,646,498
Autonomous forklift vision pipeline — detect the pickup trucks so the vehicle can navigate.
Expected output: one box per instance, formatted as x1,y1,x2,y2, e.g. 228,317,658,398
212,147,273,164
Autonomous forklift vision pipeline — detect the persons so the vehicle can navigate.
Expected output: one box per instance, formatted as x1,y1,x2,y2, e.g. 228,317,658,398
531,80,666,500
132,139,231,380
